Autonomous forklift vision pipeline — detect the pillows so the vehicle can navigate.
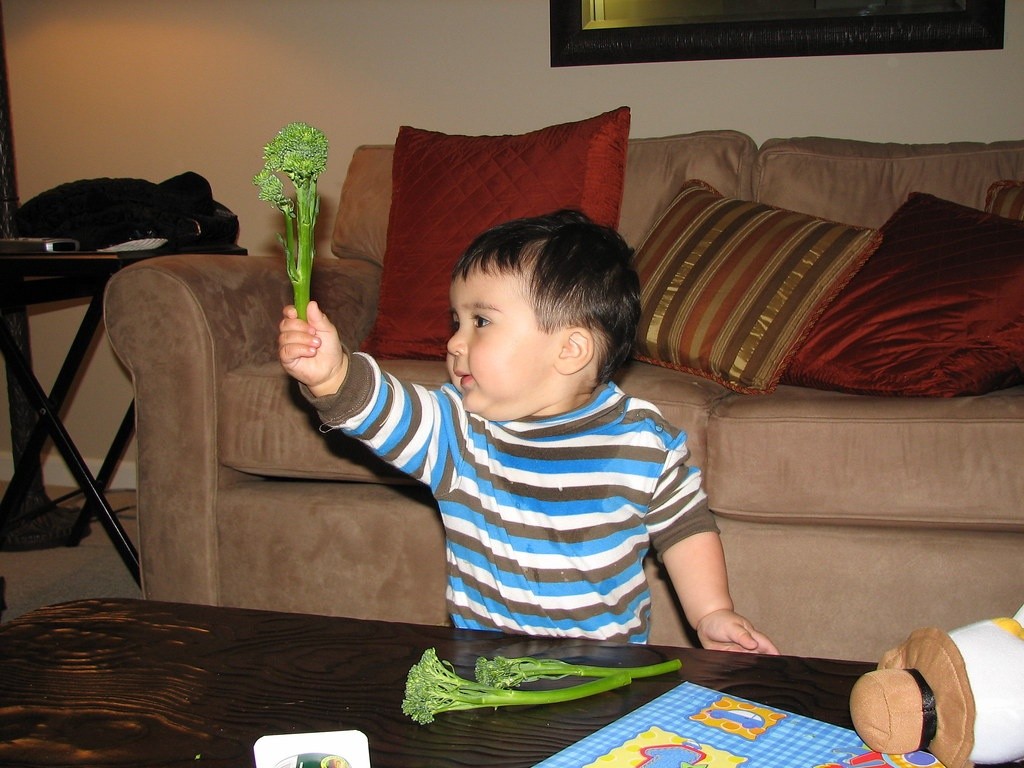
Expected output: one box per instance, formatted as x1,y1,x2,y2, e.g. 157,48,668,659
626,179,884,394
985,179,1023,222
358,106,632,362
779,191,1024,396
753,135,1024,230
331,128,757,268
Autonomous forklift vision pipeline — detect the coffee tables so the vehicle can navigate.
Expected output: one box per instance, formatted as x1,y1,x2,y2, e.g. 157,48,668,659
1,599,1024,768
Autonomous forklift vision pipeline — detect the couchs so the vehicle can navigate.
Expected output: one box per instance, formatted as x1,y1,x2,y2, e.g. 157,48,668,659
103,252,1024,665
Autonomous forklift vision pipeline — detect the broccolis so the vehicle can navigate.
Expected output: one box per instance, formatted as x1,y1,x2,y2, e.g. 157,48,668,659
253,122,329,321
402,648,682,724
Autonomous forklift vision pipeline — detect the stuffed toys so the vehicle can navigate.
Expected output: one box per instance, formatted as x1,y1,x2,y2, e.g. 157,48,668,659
848,604,1024,768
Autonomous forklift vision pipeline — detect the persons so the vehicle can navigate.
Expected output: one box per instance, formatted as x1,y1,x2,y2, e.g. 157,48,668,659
280,209,778,657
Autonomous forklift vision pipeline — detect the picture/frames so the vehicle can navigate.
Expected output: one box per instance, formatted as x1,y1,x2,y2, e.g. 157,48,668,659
549,1,1007,68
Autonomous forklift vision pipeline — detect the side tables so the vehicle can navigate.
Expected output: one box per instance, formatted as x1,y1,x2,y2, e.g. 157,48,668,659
0,245,249,592
